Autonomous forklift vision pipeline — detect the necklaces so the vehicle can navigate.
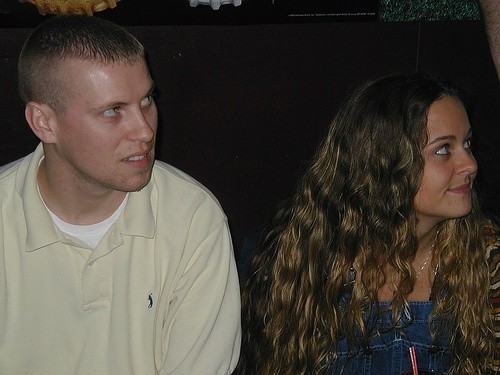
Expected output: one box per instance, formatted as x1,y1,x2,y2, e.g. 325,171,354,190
414,227,436,281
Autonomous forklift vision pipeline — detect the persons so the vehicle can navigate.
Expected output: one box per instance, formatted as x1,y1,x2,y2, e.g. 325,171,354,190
0,18,243,375
248,70,500,375
